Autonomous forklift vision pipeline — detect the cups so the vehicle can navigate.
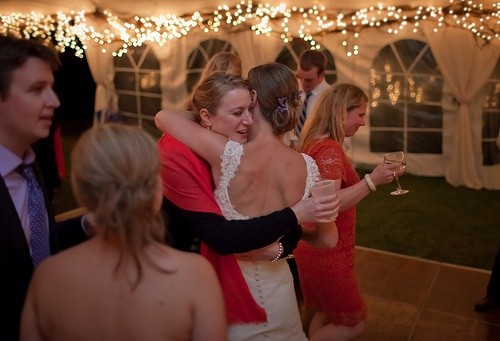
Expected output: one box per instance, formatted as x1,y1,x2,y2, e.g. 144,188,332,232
309,180,338,220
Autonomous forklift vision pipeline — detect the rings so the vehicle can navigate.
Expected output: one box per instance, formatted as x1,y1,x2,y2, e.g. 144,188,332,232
392,172,396,176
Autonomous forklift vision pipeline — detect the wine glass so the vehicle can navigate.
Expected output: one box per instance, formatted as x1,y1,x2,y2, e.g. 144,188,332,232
384,151,409,195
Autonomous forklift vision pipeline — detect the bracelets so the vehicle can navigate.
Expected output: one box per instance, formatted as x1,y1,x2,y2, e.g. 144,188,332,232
365,174,376,192
271,243,283,263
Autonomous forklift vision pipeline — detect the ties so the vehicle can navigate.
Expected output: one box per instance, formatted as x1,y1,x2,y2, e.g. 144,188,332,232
15,165,50,266
294,92,312,138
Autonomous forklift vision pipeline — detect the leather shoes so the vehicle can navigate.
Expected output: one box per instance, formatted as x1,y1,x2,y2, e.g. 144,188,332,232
475,297,497,314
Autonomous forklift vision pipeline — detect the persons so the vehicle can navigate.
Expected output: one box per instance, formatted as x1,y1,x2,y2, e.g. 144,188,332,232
20,123,228,341
283,50,352,159
293,83,407,341
475,245,500,313
154,63,339,341
198,52,243,87
0,33,60,341
150,71,341,308
